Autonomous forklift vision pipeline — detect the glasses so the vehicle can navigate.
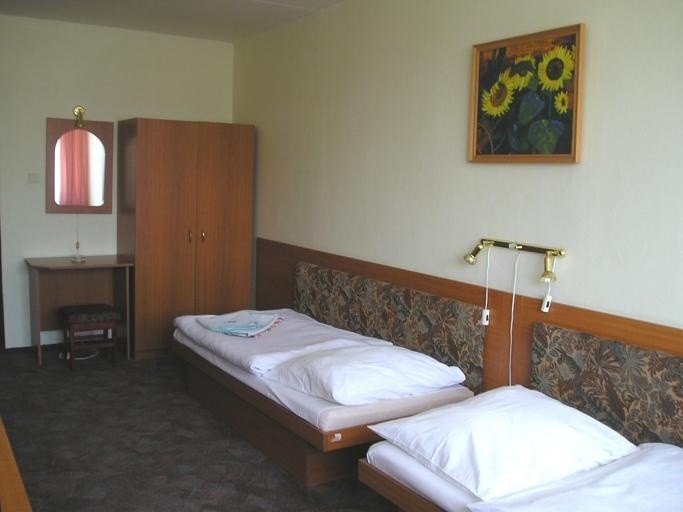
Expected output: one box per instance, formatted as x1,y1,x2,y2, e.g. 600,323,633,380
464,239,565,284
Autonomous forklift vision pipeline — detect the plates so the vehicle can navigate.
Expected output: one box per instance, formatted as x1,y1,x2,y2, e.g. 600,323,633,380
118,118,254,363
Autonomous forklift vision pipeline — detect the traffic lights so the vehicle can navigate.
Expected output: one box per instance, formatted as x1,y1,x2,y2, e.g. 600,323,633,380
264,343,467,407
367,384,637,501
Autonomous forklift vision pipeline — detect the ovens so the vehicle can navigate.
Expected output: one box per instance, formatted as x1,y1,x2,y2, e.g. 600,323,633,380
358,321,681,511
170,261,483,487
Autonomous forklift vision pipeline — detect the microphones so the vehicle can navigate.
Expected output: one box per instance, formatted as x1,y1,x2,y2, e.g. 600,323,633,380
59,304,122,368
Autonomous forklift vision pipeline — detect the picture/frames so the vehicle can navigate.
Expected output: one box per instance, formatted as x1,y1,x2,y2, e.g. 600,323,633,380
25,256,134,366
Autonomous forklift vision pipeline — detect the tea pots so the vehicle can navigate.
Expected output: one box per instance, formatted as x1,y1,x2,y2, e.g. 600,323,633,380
46,117,112,213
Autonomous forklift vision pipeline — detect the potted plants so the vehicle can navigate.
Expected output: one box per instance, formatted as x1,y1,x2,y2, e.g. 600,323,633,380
470,20,585,164
